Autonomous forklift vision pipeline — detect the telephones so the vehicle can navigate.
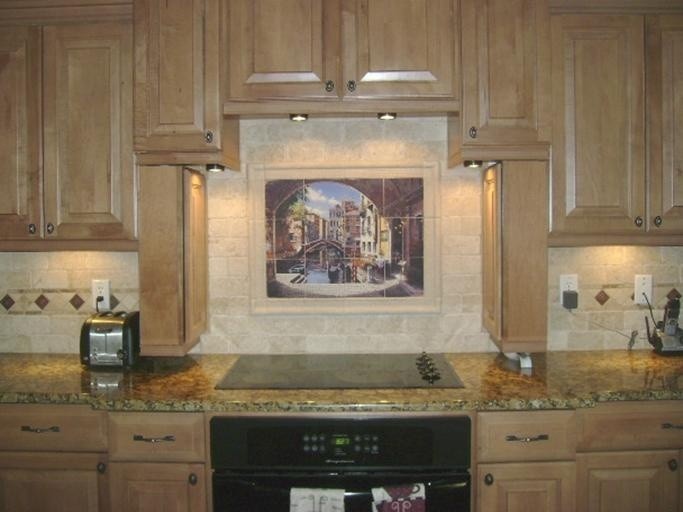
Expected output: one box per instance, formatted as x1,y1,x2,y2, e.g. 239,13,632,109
660,299,683,351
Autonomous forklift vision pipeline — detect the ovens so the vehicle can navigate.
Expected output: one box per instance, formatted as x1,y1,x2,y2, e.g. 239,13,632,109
208,414,474,512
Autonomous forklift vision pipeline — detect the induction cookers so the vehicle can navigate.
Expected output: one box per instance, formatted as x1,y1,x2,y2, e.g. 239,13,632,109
214,351,467,394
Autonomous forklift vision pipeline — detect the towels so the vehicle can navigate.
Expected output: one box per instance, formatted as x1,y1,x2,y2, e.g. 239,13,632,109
287,480,428,511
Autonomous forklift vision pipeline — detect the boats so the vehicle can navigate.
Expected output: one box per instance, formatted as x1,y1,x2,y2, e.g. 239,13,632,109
288,263,305,273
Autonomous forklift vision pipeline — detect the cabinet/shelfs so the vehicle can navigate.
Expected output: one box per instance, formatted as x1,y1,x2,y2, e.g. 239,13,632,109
135,1,239,169
550,0,683,246
138,164,210,358
472,411,578,511
447,1,551,166
222,0,461,119
576,401,683,512
1,0,139,251
0,403,209,512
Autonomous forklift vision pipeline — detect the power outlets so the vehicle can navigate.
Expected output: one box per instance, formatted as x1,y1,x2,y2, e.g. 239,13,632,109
560,273,577,305
90,279,111,311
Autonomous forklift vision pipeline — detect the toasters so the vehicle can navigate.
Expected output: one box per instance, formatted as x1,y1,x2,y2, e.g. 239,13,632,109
79,309,141,371
